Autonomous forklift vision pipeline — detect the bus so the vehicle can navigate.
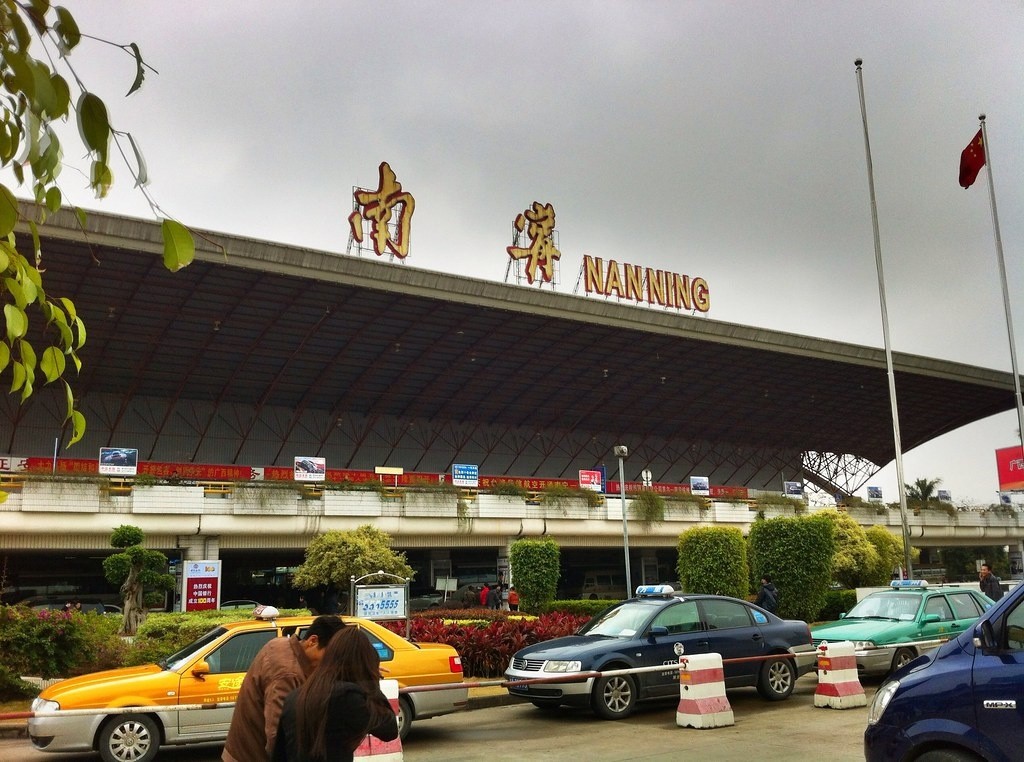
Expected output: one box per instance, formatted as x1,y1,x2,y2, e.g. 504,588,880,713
576,570,635,600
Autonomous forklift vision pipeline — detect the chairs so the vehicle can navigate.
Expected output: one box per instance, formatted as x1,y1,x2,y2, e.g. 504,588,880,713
234,636,263,670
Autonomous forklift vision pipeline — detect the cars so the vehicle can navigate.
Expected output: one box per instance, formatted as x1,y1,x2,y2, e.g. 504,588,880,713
409,583,444,613
446,583,506,609
866,582,1024,762
28,607,468,762
217,599,259,612
507,586,818,719
812,579,996,680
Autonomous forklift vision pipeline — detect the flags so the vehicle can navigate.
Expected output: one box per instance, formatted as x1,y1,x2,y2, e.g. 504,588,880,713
958,129,987,190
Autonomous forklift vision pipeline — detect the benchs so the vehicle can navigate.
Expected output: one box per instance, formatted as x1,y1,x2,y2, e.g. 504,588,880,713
706,613,749,628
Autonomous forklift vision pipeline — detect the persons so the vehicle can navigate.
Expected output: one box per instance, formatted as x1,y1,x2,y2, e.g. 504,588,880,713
494,586,504,610
61,600,72,612
979,564,1004,603
753,575,779,614
271,627,398,762
508,586,520,612
464,585,478,607
73,600,85,612
297,595,308,609
479,582,490,606
221,615,347,762
